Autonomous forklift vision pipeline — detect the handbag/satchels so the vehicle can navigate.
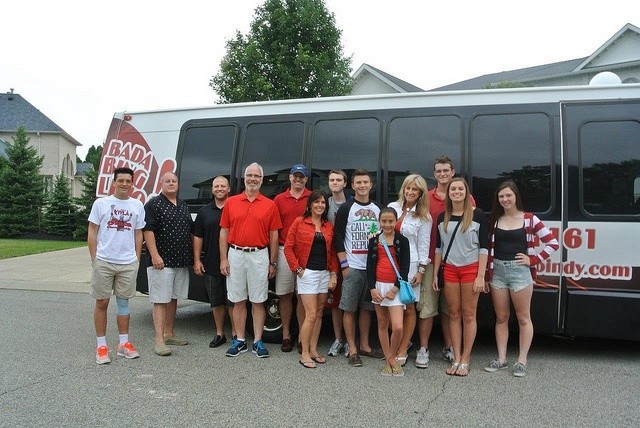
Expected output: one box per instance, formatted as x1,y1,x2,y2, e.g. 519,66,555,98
438,213,464,287
381,233,416,304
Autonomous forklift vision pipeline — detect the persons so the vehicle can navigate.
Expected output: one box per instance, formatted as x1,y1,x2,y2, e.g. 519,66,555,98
283,190,337,368
483,181,552,377
365,207,412,377
273,164,312,353
217,162,283,357
327,168,354,359
86,166,147,365
193,175,237,348
431,177,491,376
330,169,385,366
387,173,433,367
142,172,194,356
414,155,476,369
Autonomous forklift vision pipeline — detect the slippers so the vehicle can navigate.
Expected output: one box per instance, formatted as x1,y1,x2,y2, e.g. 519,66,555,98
311,350,326,363
394,354,409,365
299,360,317,368
381,362,404,376
446,362,471,376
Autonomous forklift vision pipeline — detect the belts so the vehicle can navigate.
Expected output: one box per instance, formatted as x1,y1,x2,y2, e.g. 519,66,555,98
230,244,266,252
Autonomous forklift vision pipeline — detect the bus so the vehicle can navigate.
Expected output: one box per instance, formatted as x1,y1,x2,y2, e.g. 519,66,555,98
94,83,640,343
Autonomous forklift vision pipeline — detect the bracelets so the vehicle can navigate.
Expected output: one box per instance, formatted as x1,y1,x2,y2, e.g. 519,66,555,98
339,258,349,270
296,266,302,274
416,264,426,274
390,289,396,295
270,262,278,269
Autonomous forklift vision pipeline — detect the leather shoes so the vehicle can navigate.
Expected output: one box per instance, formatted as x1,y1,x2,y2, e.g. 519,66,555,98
154,341,172,356
209,335,226,348
164,334,188,345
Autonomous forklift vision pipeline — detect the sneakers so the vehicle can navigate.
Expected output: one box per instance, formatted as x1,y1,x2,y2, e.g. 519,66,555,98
225,339,248,357
252,339,269,358
414,347,430,368
441,345,455,364
117,342,139,359
357,343,386,360
296,339,302,355
349,351,362,367
344,342,349,357
483,358,510,372
96,346,111,364
512,361,530,376
280,331,294,352
326,338,345,357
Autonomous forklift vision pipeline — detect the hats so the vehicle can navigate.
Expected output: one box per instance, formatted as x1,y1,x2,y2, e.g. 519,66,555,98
289,163,309,177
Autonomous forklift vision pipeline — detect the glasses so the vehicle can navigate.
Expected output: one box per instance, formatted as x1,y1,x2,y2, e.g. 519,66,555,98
246,174,262,178
